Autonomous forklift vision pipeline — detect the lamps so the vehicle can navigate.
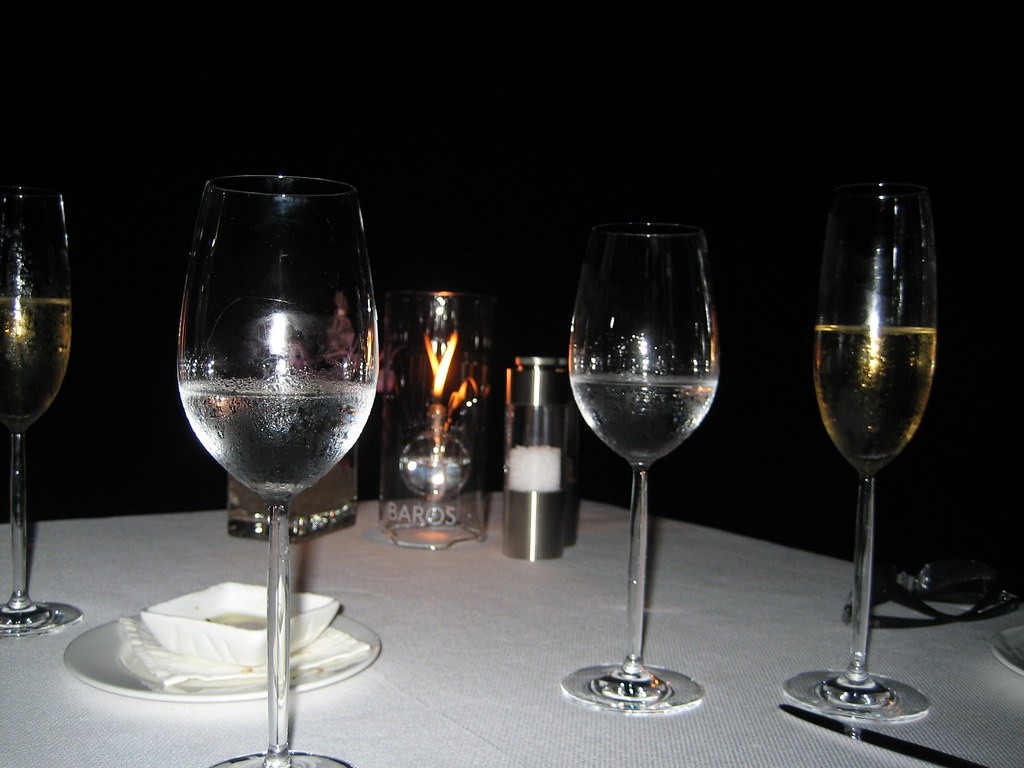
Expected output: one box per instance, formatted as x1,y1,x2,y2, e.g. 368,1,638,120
379,291,493,551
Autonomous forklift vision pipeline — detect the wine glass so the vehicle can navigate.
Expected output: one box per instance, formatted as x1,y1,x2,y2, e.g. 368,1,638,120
176,173,380,768
782,179,936,725
560,227,720,717
0,184,83,639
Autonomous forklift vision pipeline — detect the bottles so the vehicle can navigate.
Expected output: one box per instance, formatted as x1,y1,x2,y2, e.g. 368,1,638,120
504,354,561,561
555,357,581,546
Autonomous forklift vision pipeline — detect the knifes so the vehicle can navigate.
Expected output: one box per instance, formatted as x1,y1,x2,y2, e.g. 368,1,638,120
777,701,986,768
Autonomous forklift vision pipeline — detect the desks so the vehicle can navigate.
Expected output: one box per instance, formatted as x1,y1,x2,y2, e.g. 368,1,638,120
1,495,1024,767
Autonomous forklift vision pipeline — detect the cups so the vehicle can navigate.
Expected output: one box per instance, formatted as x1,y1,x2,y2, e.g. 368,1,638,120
380,289,488,552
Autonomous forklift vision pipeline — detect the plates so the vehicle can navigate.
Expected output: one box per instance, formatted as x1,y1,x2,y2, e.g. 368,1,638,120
62,600,383,702
990,624,1024,677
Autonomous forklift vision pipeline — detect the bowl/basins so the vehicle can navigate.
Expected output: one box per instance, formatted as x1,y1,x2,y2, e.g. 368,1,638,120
139,581,340,664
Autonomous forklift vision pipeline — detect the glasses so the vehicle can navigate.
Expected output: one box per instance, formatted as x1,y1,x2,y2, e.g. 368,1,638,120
842,555,1018,630
